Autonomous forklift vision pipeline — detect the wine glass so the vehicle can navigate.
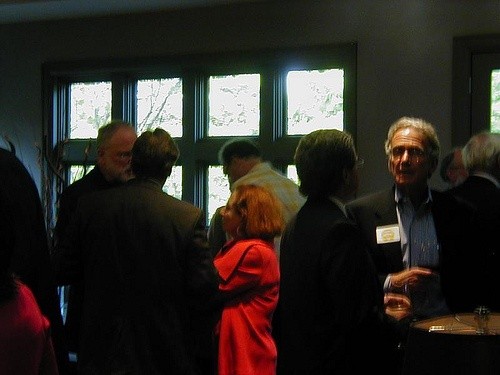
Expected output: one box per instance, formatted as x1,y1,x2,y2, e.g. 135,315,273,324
385,278,413,350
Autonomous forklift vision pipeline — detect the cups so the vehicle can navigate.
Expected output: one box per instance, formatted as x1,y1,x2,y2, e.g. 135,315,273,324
474,307,493,335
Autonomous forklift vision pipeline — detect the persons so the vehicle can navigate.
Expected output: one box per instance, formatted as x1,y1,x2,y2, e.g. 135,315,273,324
52,121,139,375
0,148,58,375
67,128,219,375
438,147,471,190
442,132,500,311
219,140,306,322
213,184,285,374
345,117,485,374
279,130,412,375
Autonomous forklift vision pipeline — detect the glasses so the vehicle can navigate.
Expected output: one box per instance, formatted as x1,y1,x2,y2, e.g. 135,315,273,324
352,158,364,169
